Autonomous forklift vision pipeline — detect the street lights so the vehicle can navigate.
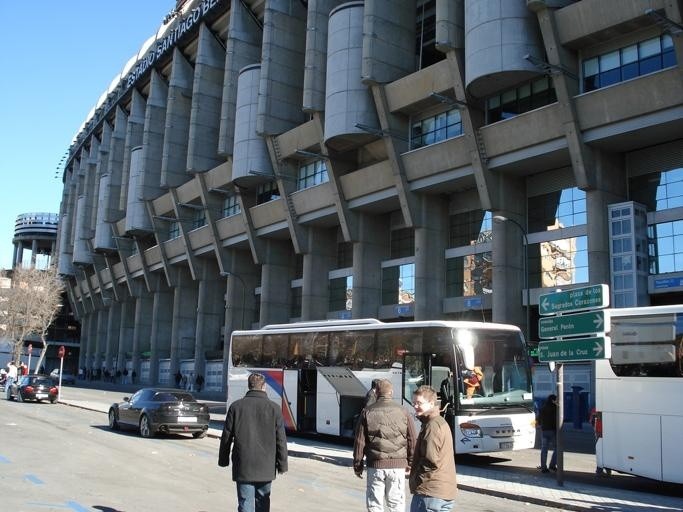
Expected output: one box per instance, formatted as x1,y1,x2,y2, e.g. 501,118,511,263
489,212,533,358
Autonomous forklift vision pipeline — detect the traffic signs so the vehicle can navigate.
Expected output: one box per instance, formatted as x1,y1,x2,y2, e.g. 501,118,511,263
538,282,612,317
537,309,612,340
535,335,613,364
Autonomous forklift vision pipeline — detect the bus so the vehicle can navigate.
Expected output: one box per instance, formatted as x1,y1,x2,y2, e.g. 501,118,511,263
590,300,683,492
222,312,537,462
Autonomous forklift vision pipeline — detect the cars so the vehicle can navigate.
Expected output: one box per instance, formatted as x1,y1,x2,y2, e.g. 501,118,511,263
49,367,76,386
6,373,58,404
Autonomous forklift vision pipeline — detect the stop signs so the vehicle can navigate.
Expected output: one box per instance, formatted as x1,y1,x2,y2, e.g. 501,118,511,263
27,344,34,355
56,345,66,359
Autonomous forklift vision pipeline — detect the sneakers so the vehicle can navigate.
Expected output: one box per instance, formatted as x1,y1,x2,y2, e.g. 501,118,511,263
549,462,558,470
541,468,550,473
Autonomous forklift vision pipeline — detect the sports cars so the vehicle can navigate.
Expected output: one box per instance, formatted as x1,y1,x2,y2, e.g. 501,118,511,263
106,386,211,442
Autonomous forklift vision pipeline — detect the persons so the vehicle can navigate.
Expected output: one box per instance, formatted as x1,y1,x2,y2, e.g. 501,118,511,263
217,372,287,511
169,370,204,394
2,359,18,392
76,364,136,386
0,368,8,392
351,379,416,512
537,393,556,474
361,379,382,409
408,384,458,512
15,360,28,376
